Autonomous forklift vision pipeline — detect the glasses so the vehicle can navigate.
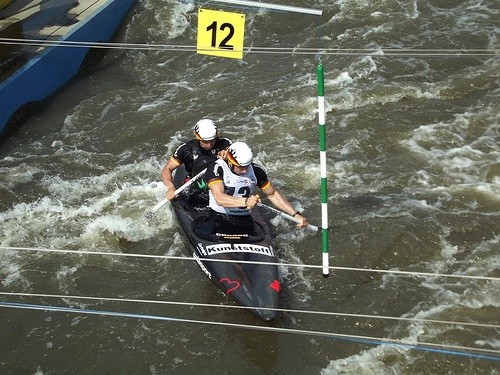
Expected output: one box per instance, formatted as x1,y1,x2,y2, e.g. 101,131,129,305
202,139,214,144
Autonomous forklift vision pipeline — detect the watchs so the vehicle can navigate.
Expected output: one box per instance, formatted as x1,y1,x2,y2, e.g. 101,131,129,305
293,211,302,217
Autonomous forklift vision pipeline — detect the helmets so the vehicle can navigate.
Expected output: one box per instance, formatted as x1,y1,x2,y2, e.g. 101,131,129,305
226,141,254,168
195,118,218,141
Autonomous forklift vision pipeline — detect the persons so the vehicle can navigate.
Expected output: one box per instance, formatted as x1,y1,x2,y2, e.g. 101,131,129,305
162,119,233,199
205,142,308,233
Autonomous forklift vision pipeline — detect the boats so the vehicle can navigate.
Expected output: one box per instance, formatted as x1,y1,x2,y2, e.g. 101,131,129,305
172,165,280,322
0,0,138,141
